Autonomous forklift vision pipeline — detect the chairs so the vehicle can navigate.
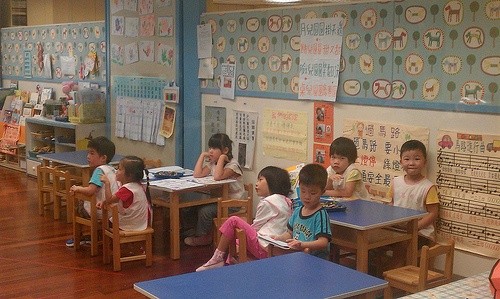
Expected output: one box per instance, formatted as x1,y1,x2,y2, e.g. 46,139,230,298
37,166,155,272
380,240,455,299
214,182,310,265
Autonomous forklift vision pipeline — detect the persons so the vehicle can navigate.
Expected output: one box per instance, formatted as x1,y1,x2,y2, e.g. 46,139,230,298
322,137,369,199
315,152,323,163
66,136,121,246
184,133,245,245
317,127,323,136
96,155,153,255
376,140,439,274
316,108,324,119
271,163,332,261
195,166,294,272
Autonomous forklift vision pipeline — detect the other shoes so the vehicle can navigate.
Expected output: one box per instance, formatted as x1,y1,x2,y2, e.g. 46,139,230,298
66,237,86,247
195,260,224,272
84,235,91,244
184,235,212,246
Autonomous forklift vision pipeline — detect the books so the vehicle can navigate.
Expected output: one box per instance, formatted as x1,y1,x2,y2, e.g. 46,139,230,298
258,236,298,251
3,88,52,126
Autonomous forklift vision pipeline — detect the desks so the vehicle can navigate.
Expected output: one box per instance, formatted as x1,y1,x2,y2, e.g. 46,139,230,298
329,199,430,273
398,270,493,299
134,252,390,299
142,175,236,258
36,151,122,210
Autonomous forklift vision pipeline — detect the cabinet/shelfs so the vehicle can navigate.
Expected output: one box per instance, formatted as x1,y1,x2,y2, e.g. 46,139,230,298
25,117,108,185
